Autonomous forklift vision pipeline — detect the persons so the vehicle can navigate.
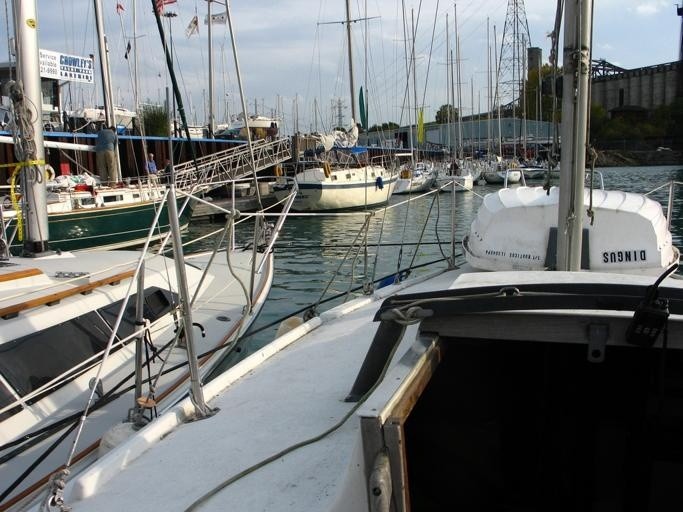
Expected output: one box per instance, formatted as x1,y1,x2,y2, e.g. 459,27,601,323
95,126,118,186
162,156,173,182
143,153,157,175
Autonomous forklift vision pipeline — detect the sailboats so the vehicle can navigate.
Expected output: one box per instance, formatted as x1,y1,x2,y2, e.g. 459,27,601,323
38,1,681,510
1,1,299,503
0,0,565,253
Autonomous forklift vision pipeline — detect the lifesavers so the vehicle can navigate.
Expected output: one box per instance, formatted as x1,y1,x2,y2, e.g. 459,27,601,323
324,162,332,178
43,164,55,180
276,166,282,176
